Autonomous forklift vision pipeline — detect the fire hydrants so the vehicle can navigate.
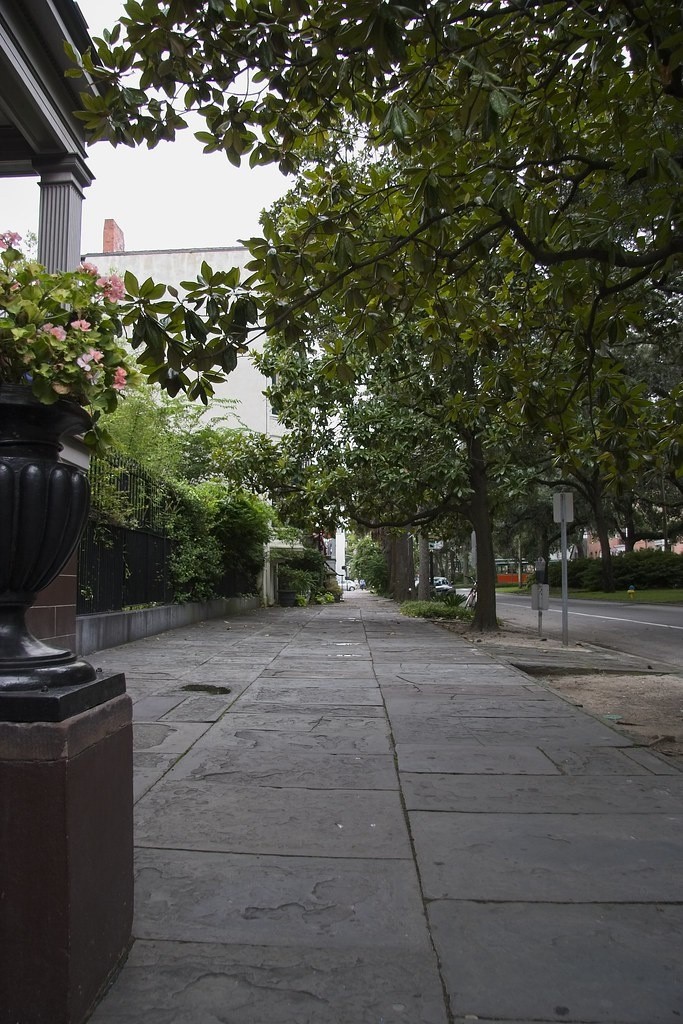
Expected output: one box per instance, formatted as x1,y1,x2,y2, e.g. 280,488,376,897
628,586,635,597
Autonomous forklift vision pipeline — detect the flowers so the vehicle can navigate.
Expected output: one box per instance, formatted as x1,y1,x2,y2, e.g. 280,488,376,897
0,234,141,460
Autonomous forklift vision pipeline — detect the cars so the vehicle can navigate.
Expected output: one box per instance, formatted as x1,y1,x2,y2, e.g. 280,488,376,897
346,579,358,591
433,576,456,597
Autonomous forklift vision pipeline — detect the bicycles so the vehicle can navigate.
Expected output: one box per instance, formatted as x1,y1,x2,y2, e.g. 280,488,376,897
463,576,478,609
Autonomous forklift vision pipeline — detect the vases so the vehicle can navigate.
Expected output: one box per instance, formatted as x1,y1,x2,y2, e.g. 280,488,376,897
277,590,297,607
0,386,127,723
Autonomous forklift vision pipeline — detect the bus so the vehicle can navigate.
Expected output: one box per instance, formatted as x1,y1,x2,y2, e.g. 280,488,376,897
495,557,560,587
